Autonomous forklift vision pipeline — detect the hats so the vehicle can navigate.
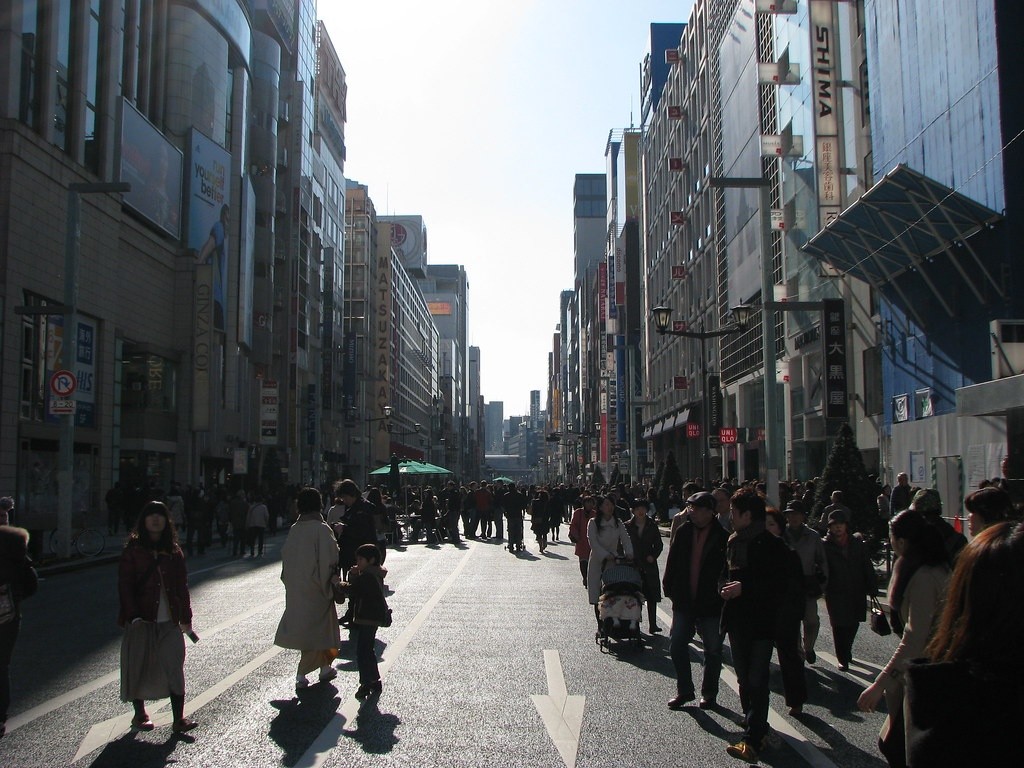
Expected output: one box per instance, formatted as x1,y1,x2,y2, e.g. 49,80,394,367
686,491,717,511
0,496,15,525
783,500,807,512
825,510,851,528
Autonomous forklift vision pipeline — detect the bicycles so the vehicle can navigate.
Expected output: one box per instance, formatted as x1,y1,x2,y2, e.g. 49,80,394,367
869,514,897,566
50,509,106,558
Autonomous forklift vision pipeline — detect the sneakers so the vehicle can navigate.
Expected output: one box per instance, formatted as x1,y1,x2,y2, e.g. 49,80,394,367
726,742,759,764
759,735,769,754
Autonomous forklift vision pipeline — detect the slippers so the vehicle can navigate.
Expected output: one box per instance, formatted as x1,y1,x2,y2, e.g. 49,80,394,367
319,666,337,680
296,676,309,689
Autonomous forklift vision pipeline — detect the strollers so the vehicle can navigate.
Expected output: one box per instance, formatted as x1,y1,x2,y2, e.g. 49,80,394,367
504,512,525,551
595,556,642,654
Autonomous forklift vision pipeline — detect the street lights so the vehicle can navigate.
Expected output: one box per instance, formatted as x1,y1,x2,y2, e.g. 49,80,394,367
345,402,392,485
14,182,131,559
652,297,752,491
387,421,422,458
708,178,825,512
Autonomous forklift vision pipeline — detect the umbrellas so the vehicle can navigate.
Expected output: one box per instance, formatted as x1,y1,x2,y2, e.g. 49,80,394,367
411,457,453,505
367,455,441,513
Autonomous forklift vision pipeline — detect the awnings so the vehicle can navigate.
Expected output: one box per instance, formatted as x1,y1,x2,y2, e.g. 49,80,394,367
642,409,692,440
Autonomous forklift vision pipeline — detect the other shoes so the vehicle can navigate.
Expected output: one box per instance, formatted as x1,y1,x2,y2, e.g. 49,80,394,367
788,705,802,715
0,722,6,736
132,715,155,730
838,660,847,670
355,681,382,701
172,719,198,734
649,623,662,633
805,650,816,664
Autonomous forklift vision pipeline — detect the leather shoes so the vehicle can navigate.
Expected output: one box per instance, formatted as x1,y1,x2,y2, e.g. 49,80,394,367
700,696,716,708
667,693,695,707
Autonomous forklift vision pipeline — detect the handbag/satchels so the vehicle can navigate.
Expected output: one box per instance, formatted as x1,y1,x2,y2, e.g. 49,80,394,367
0,584,16,625
568,531,577,543
353,603,392,627
871,596,891,636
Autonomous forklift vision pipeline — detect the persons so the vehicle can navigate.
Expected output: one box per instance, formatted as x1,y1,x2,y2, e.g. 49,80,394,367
105,453,1024,556
333,480,375,631
570,495,599,585
624,498,663,633
662,492,731,708
759,506,786,537
530,486,549,554
856,509,953,768
588,494,635,641
331,544,391,699
783,500,829,665
964,485,1020,538
274,488,340,685
720,486,790,763
501,483,526,552
550,486,564,543
824,490,849,515
118,500,198,733
599,588,644,630
900,522,1024,768
823,510,878,672
0,496,38,741
